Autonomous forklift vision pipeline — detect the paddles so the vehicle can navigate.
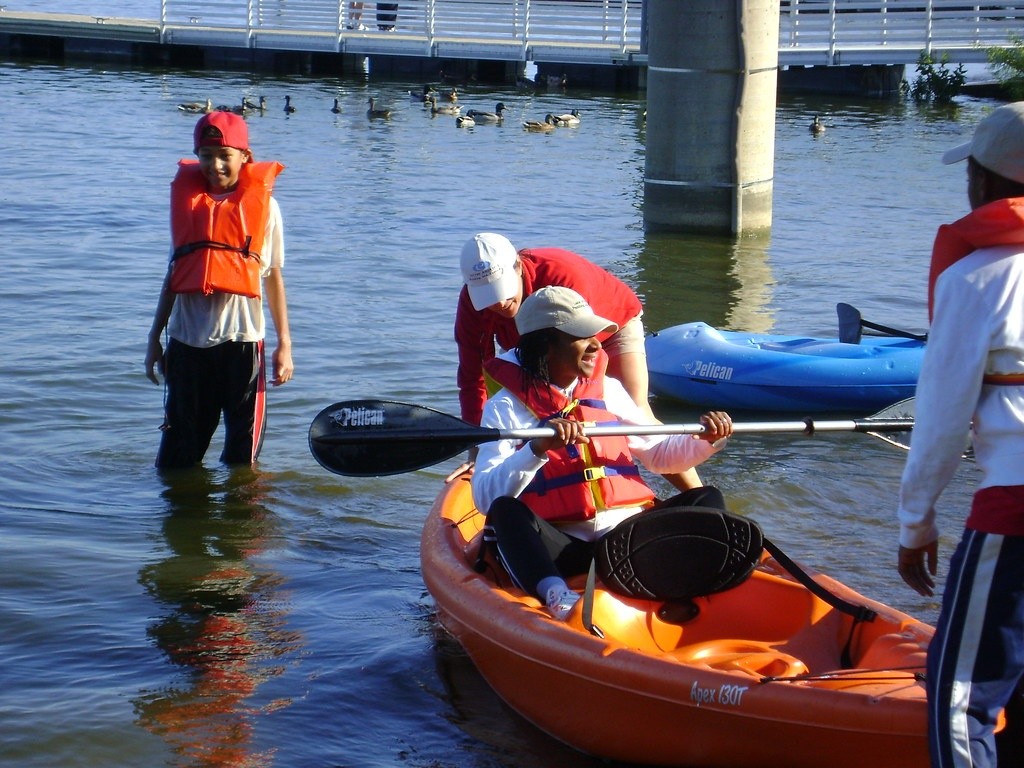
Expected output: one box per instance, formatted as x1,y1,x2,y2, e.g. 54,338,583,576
833,299,928,347
305,396,974,479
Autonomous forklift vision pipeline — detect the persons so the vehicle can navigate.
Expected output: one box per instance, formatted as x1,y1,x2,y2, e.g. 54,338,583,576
454,233,735,621
144,112,293,467
345,3,399,32
131,474,296,768
897,98,1024,768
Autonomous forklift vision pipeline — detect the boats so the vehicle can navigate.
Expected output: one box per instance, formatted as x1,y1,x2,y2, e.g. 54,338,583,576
419,459,1008,767
646,301,932,414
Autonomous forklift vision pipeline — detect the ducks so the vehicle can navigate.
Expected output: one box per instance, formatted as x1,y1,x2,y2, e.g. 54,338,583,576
809,116,827,133
522,109,581,134
366,97,391,119
408,84,464,116
176,95,298,117
456,103,509,127
331,99,342,114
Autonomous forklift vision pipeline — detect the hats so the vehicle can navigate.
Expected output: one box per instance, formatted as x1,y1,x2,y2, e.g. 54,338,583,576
459,233,519,311
942,101,1024,185
514,285,619,338
192,112,253,163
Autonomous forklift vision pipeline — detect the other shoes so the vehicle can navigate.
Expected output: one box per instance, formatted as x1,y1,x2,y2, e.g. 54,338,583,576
544,584,581,621
